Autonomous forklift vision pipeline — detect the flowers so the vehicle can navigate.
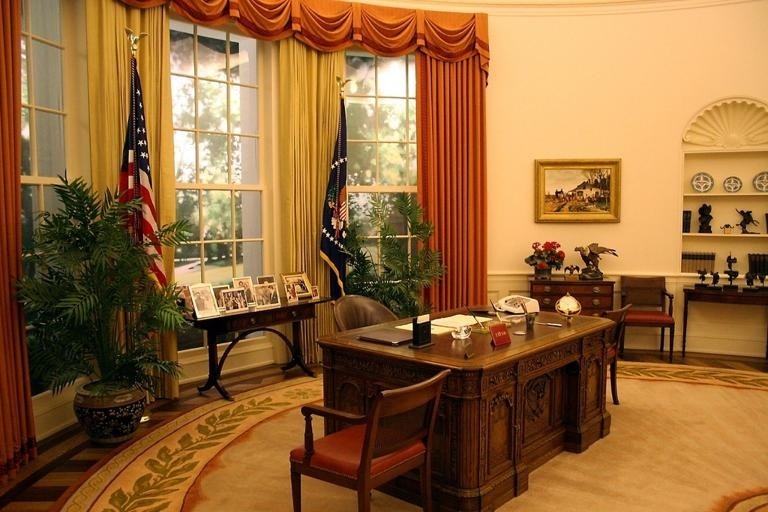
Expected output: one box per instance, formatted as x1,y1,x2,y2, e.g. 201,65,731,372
525,241,565,271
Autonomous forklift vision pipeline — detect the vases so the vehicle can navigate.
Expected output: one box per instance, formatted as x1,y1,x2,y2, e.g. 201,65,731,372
536,266,552,279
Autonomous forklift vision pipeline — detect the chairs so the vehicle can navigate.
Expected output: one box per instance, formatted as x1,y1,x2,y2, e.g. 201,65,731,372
332,294,399,331
600,303,633,406
289,368,453,512
618,275,675,363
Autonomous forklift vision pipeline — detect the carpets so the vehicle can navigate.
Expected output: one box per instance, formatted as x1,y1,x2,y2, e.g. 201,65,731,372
51,358,768,512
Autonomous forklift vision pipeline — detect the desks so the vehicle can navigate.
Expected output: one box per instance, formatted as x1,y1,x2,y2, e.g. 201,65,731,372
314,301,616,512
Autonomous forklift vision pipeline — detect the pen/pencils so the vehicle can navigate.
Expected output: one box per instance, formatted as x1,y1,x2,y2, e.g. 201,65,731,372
519,299,528,314
538,322,562,327
490,299,501,322
463,303,483,328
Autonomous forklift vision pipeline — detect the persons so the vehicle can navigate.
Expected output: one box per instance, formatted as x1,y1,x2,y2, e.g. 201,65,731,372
288,284,297,300
195,291,204,311
217,290,223,308
244,282,252,302
202,290,213,309
268,285,279,304
239,281,243,287
226,291,234,311
233,292,246,308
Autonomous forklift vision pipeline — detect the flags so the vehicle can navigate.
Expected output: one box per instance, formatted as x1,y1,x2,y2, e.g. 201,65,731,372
116,59,166,300
319,97,348,300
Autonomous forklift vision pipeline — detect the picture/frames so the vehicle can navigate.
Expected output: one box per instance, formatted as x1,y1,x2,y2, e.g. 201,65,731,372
232,276,255,305
212,285,229,311
311,286,319,300
279,271,312,299
284,283,298,303
256,275,275,284
221,288,249,314
253,283,281,309
189,284,220,318
533,158,623,224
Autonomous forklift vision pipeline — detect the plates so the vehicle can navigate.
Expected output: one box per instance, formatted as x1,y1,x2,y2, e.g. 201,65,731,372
722,176,742,193
751,171,767,193
690,172,713,193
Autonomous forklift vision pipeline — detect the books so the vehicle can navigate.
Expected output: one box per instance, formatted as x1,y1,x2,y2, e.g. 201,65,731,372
427,313,493,329
394,322,456,335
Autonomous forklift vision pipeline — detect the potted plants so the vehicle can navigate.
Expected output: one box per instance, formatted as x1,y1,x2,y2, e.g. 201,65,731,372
12,170,193,444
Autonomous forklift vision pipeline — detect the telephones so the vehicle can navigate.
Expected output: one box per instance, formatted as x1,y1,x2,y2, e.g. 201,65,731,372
493,295,541,314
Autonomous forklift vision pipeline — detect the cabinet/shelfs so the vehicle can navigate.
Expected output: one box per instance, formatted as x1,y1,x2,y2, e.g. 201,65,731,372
680,151,768,276
682,285,768,358
192,297,331,402
529,277,615,318
